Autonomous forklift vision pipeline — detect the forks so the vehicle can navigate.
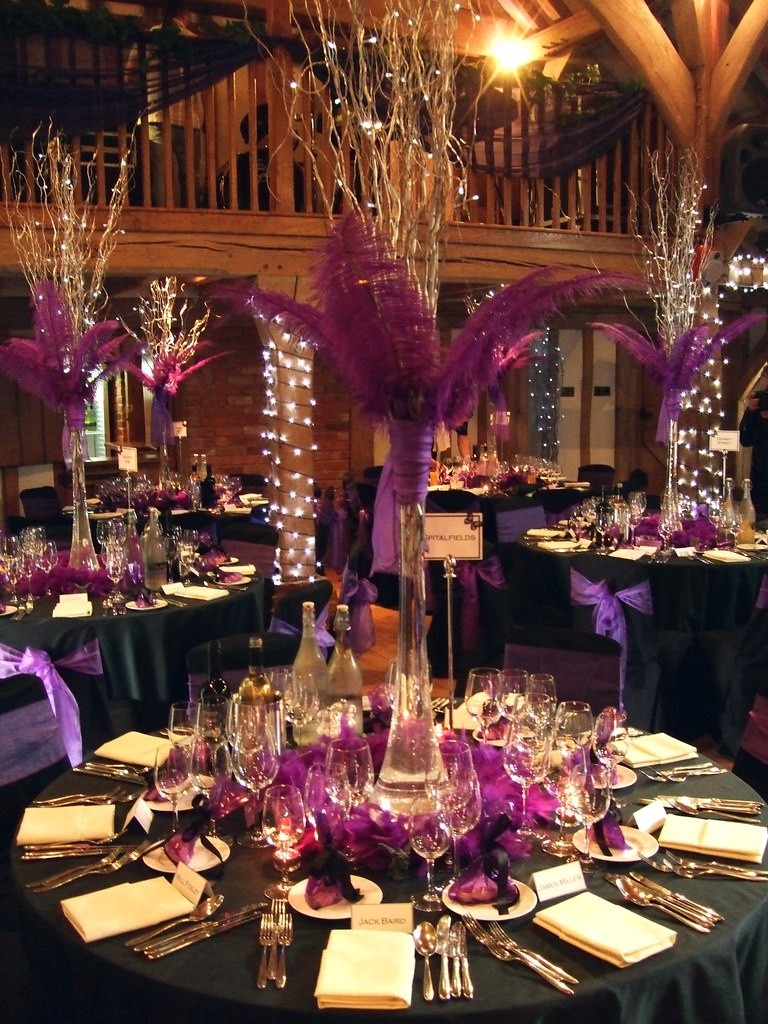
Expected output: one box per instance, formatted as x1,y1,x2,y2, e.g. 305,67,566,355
589,726,726,790
574,795,767,932
413,875,577,1002
19,759,381,989
431,690,525,730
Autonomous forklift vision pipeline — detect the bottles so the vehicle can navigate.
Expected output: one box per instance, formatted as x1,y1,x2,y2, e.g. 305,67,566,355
594,485,613,548
610,483,630,542
200,641,232,775
324,605,363,739
290,602,330,747
716,477,756,552
240,637,273,705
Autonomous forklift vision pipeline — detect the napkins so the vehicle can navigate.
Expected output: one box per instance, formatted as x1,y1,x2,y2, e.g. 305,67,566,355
52,602,93,618
538,541,580,551
16,804,116,846
87,498,100,505
218,564,257,575
658,813,768,864
611,732,699,769
312,928,416,1010
239,495,269,507
60,876,196,944
88,512,123,520
445,691,524,731
607,549,646,560
564,481,591,488
702,549,752,563
224,507,252,515
174,585,230,601
527,529,564,537
533,891,678,968
99,734,172,767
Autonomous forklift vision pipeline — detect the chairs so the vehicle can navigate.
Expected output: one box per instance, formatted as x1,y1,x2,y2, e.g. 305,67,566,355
0,671,116,933
185,631,302,722
365,466,383,487
269,579,336,661
715,573,768,763
731,688,768,803
19,486,60,519
495,497,547,565
570,554,666,733
454,540,528,679
218,521,279,579
426,491,482,616
315,486,348,576
356,482,399,608
577,464,616,496
325,538,378,664
502,624,623,715
229,472,268,494
6,514,71,540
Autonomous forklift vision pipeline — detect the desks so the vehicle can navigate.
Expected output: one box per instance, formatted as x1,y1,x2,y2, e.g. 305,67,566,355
0,551,266,733
0,695,768,1024
65,499,270,538
511,507,768,761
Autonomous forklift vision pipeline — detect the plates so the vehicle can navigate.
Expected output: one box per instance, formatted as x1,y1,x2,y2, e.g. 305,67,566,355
736,543,768,551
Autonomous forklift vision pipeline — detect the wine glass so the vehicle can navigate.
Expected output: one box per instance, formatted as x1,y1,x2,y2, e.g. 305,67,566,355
0,510,267,613
154,668,627,912
569,491,647,552
446,454,562,500
88,453,267,514
